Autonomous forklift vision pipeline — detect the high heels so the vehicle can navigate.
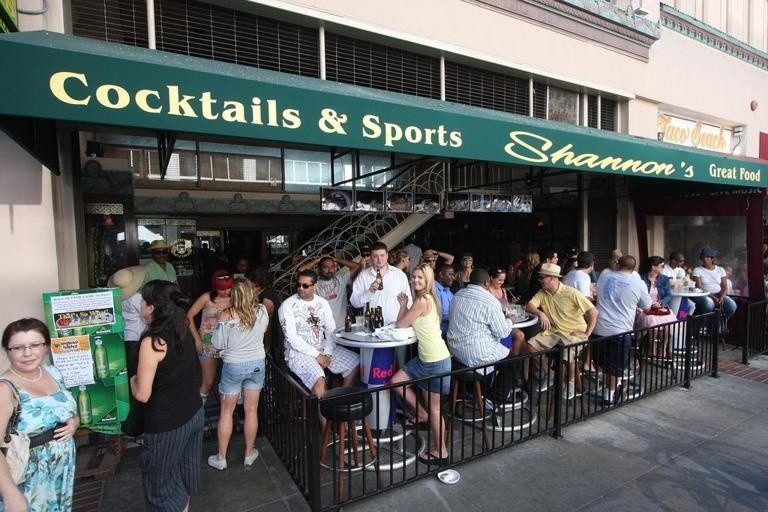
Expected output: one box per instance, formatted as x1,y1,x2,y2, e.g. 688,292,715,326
400,417,429,431
418,449,450,466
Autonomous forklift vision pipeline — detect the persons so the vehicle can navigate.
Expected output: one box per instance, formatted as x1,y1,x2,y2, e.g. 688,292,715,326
526,261,599,400
145,240,177,283
351,242,411,323
397,263,449,466
449,252,474,295
198,242,232,268
692,247,737,336
105,265,149,442
486,264,525,357
446,269,515,414
1,319,80,512
664,251,696,316
419,249,453,279
248,278,278,354
562,251,597,373
641,256,677,359
187,270,233,407
279,270,360,442
595,256,651,402
353,244,411,270
230,258,255,281
299,253,360,331
432,264,455,348
723,242,768,299
207,279,269,471
131,280,204,512
559,252,579,283
507,240,557,305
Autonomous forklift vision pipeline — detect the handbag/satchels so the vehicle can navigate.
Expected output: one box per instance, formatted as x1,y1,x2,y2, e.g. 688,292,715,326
644,305,671,316
0,428,32,488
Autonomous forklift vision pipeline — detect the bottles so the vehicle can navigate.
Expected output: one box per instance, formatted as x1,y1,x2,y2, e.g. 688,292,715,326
94,337,110,379
369,308,374,328
365,302,369,326
375,269,383,290
375,308,381,328
379,307,384,327
345,307,351,331
377,306,380,308
76,384,92,424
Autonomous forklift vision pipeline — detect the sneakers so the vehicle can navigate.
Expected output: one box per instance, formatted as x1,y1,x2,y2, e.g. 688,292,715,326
243,448,259,468
535,369,555,393
600,400,614,408
207,454,228,471
562,382,575,401
481,392,503,417
614,385,627,406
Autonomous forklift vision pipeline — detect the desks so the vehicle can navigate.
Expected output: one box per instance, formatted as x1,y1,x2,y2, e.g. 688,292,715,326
457,307,539,432
332,321,426,473
73,422,122,480
654,288,709,370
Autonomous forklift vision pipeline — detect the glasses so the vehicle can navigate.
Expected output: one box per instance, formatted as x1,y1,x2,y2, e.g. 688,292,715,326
6,341,47,353
213,273,233,282
294,281,315,289
490,267,506,274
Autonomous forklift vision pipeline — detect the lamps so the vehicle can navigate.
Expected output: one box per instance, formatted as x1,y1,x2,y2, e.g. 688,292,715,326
618,5,648,19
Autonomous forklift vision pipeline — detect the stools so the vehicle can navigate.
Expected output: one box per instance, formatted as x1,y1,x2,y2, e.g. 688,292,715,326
319,386,383,512
590,333,644,403
702,305,728,351
536,348,590,422
452,366,500,453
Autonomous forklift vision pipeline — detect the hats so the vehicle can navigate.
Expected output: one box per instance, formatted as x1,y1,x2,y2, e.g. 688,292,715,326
146,239,170,252
699,247,716,259
536,262,564,279
106,264,146,302
420,250,438,258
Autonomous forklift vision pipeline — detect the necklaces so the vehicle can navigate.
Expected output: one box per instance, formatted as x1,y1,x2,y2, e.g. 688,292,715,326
8,365,42,382
